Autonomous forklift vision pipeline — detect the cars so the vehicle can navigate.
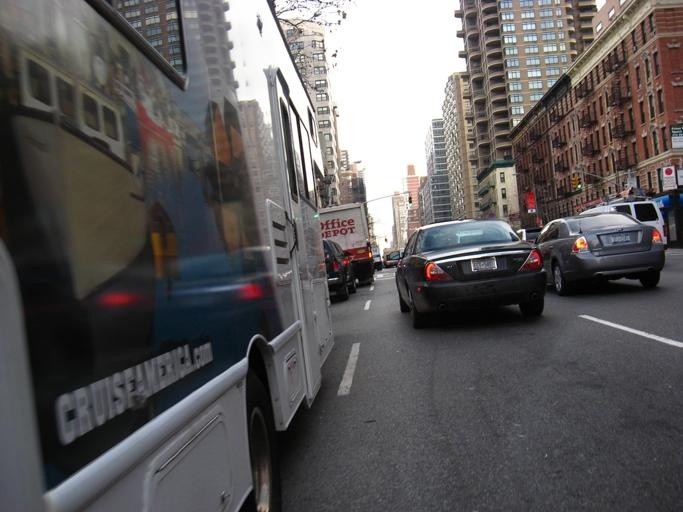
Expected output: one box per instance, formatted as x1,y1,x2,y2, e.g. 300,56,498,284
322,240,356,300
387,219,547,326
533,211,665,296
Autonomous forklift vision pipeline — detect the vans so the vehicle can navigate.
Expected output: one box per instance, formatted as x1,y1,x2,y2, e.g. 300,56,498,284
579,201,668,252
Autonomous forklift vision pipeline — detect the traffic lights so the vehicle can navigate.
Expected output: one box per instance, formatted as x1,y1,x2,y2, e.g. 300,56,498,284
408,197,412,208
572,176,582,190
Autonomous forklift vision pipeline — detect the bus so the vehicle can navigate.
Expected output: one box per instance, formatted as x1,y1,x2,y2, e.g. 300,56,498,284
0,1,334,512
382,249,400,267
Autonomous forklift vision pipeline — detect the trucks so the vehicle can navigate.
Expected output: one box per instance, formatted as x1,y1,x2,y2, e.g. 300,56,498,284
319,201,374,286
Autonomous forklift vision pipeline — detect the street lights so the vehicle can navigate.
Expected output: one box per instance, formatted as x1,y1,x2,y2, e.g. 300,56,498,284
515,173,539,218
